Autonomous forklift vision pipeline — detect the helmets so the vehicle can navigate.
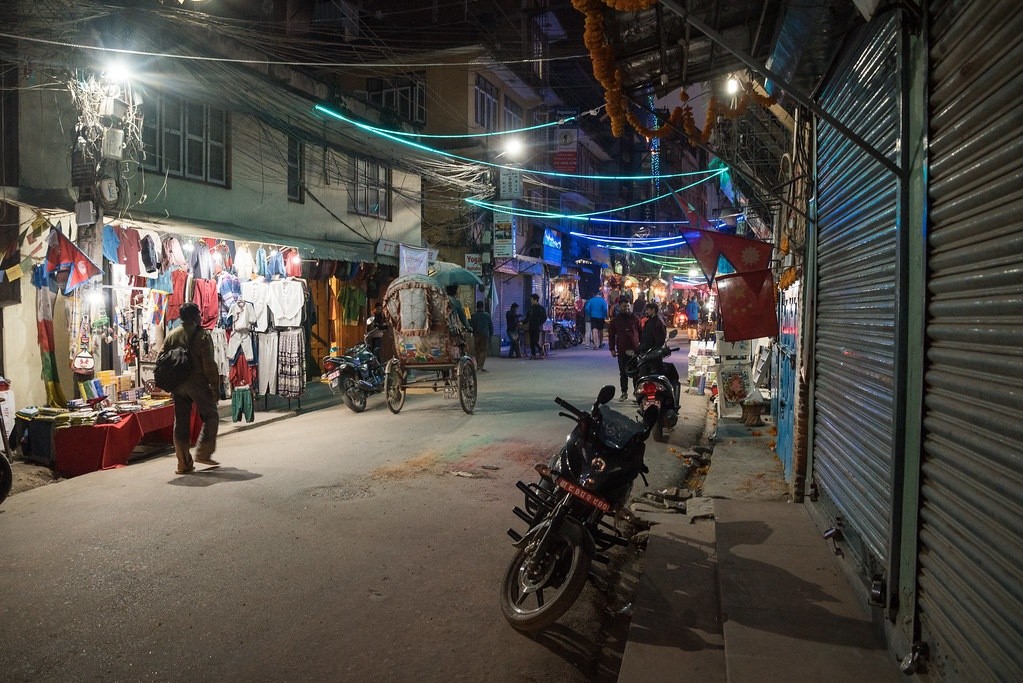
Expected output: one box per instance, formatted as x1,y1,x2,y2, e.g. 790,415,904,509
625,356,639,378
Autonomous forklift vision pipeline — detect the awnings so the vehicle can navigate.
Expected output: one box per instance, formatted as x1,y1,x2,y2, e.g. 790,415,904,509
573,0,906,223
104,209,401,265
494,257,543,275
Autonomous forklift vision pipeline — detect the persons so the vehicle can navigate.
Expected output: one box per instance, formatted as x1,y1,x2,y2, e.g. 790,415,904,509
157,304,222,472
446,285,473,333
576,285,721,350
638,303,666,353
506,304,524,358
371,303,388,330
609,299,644,401
468,301,493,372
519,294,546,360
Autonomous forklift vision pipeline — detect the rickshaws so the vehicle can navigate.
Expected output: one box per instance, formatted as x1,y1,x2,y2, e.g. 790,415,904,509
383,273,478,414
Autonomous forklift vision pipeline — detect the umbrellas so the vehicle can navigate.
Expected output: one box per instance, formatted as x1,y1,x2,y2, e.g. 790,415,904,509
429,261,484,286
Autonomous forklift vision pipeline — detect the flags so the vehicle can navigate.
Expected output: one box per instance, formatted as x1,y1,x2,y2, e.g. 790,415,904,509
715,269,779,342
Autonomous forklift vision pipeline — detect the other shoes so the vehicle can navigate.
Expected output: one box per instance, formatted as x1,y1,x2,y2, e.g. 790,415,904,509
594,346,599,350
540,354,546,358
194,459,219,465
175,467,195,474
477,366,486,371
585,346,589,348
529,355,536,359
619,392,628,402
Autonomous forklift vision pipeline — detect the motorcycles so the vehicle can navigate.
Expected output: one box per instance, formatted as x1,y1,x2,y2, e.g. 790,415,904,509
625,328,681,443
497,384,660,633
322,316,385,413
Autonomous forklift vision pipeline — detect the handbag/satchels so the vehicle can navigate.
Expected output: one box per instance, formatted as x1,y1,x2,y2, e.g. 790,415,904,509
153,326,201,393
508,329,519,342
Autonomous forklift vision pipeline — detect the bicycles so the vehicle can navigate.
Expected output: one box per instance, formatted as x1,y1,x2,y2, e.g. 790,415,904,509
553,310,583,348
